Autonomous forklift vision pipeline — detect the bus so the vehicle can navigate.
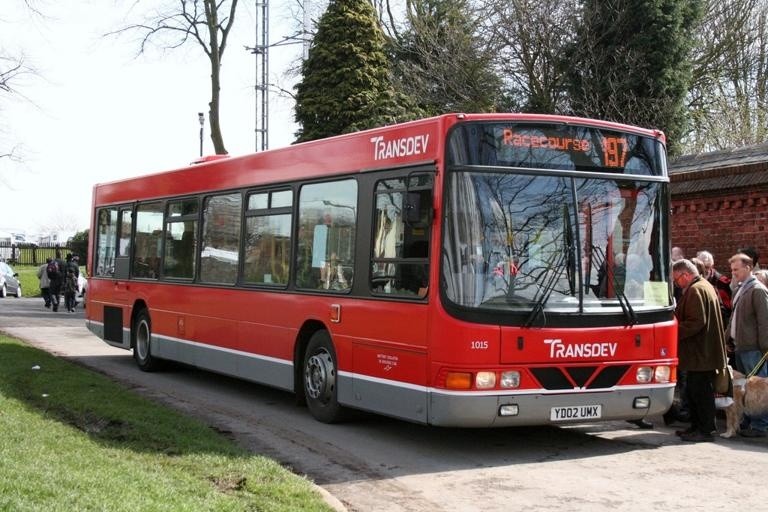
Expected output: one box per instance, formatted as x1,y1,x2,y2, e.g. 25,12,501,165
79,115,687,440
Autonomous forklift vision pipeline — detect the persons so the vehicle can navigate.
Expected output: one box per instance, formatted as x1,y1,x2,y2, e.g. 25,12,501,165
36,254,87,313
670,245,768,442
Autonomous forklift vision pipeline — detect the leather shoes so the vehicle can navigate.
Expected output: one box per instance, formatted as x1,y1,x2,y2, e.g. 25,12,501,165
627,418,654,430
675,427,715,442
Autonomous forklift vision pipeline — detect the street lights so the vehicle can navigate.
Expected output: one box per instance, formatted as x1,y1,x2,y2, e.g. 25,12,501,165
194,112,207,156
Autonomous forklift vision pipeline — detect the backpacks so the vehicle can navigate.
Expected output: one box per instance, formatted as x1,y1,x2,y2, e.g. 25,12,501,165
46,260,60,280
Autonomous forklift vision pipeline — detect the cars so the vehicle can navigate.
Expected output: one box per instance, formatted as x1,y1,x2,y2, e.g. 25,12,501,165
0,261,23,298
14,235,40,247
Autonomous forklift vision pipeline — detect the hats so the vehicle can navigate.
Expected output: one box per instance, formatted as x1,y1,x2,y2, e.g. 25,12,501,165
64,253,74,260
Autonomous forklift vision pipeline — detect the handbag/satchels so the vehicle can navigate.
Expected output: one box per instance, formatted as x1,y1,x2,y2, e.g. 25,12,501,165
713,366,729,393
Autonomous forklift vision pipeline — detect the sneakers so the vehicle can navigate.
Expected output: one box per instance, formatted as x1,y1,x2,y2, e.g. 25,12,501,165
44,302,75,313
740,427,768,438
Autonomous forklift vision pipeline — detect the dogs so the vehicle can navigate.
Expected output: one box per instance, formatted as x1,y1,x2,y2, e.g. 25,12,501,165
719,370,768,438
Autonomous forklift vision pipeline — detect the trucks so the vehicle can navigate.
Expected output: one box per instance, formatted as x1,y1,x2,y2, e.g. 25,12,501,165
0,231,20,263
